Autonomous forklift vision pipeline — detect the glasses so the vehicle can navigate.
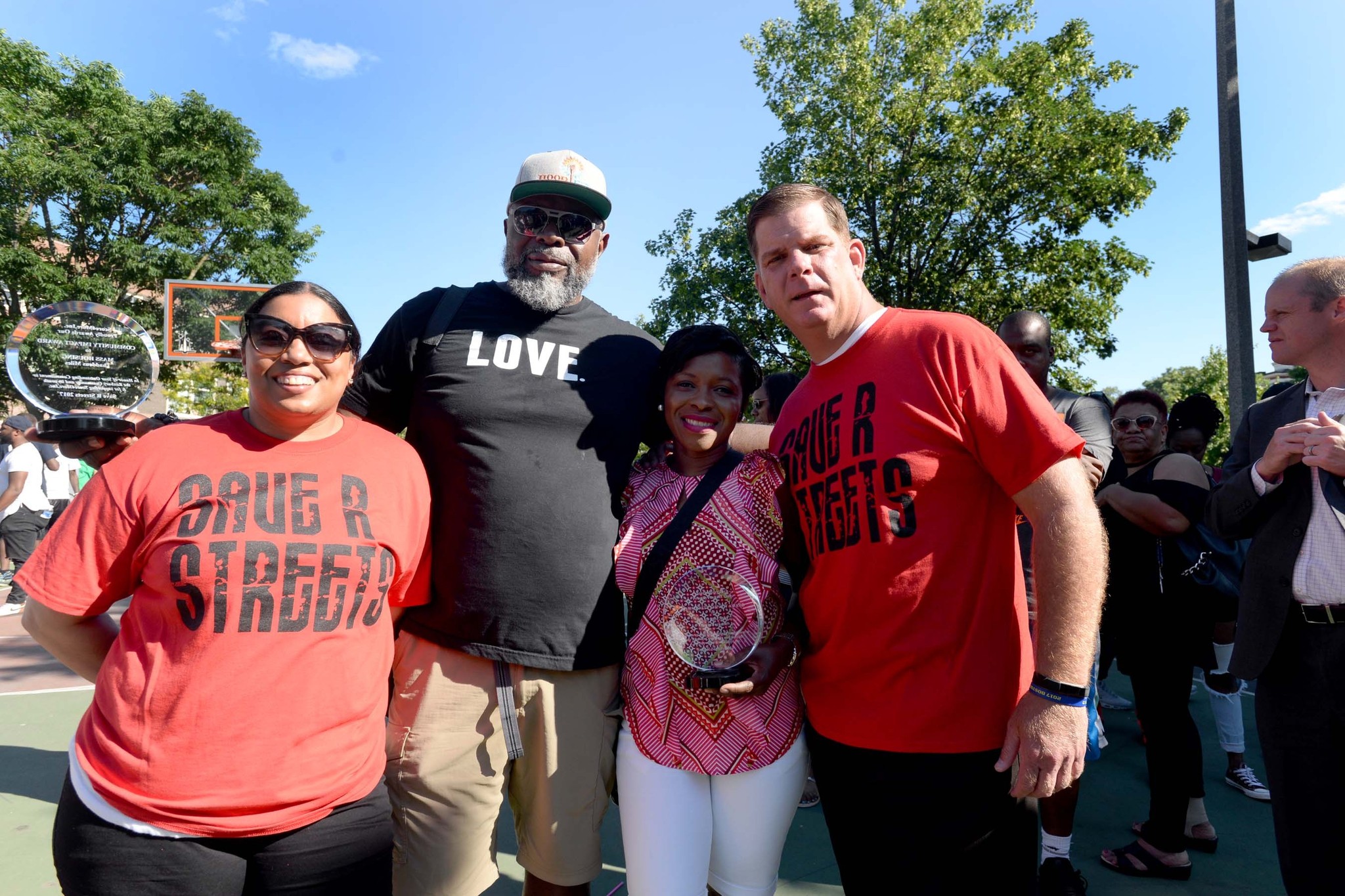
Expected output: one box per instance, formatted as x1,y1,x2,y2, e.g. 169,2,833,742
1110,415,1165,431
243,313,353,363
509,206,606,245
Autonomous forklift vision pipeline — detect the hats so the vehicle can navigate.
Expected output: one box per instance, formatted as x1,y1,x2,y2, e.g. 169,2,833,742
4,415,33,431
510,150,612,221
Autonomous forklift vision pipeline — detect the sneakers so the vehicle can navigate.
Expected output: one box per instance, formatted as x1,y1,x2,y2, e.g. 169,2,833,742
1096,680,1133,709
0,600,26,615
1224,766,1270,800
0,571,15,583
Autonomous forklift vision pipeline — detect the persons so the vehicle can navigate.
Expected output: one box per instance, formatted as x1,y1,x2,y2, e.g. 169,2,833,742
744,184,1111,896
0,412,182,615
1217,266,1345,896
615,325,812,896
133,149,787,896
21,280,432,896
991,311,1298,895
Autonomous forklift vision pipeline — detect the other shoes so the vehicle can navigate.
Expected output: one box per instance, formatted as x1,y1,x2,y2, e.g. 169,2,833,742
1036,857,1087,896
798,777,820,807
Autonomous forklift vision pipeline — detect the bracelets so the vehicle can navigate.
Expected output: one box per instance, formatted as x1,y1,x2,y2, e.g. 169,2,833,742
771,630,805,668
1028,674,1089,708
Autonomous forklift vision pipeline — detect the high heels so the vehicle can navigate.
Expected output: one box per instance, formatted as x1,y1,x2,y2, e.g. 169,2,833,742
1101,840,1191,880
1132,817,1218,855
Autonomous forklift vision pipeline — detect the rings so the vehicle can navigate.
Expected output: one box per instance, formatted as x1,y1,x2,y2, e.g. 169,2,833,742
1308,445,1317,455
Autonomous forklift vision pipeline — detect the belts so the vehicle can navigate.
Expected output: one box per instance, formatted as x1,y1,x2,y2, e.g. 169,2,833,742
1287,604,1345,624
